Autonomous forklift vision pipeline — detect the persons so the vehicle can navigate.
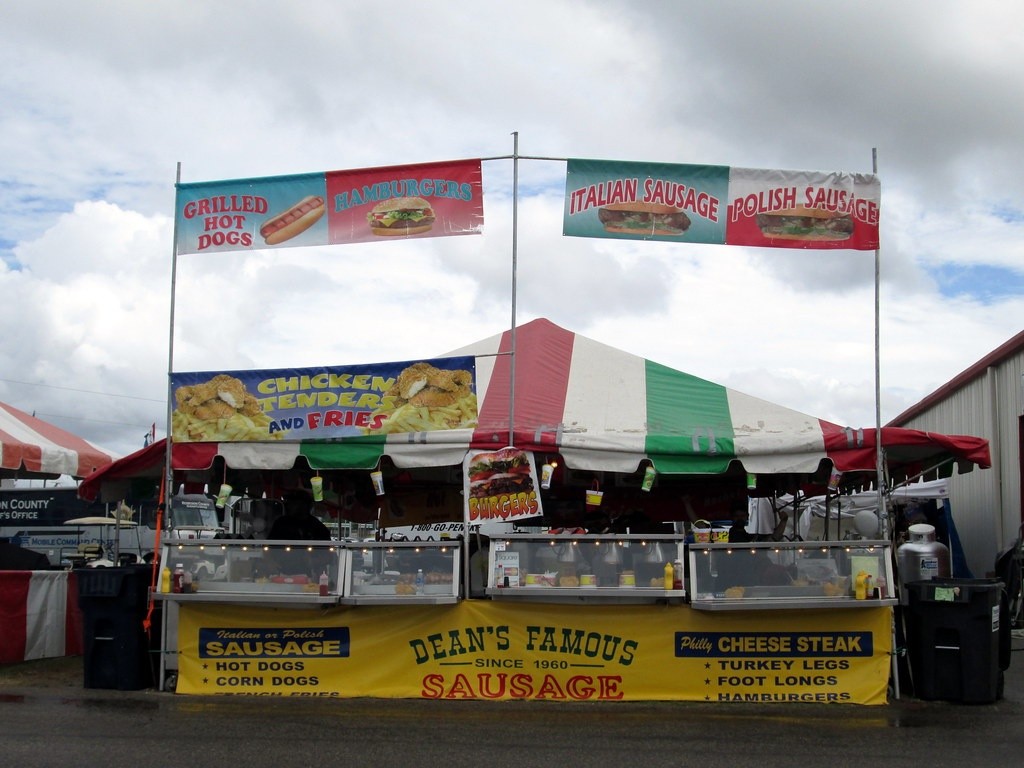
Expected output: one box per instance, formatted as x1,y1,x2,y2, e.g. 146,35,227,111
728,509,788,542
268,489,331,550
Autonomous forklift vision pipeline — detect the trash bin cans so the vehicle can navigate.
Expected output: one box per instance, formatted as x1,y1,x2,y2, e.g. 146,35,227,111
905,576,1011,703
71,562,165,688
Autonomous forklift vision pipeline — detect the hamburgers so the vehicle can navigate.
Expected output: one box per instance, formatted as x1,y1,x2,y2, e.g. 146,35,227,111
365,197,436,236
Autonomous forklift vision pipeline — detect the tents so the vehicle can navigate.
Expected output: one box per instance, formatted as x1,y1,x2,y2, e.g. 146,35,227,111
79,324,993,580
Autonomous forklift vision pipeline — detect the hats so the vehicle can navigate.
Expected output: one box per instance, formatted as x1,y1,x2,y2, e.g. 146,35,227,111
286,491,312,503
390,470,416,487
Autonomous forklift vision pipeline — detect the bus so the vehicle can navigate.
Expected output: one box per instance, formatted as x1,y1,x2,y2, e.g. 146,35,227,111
0,487,224,567
362,538,395,569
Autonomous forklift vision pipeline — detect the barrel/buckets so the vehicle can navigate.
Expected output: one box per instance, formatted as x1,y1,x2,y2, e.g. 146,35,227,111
692,519,711,543
585,478,603,505
526,574,595,587
711,528,730,543
619,575,635,587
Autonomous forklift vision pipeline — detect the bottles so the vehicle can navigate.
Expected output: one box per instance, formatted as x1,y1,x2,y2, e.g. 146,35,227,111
161,566,171,593
664,562,673,590
173,563,184,592
867,575,874,599
497,565,504,588
855,569,868,600
897,523,952,605
416,569,424,595
877,575,886,598
184,572,192,593
673,559,683,589
319,570,329,596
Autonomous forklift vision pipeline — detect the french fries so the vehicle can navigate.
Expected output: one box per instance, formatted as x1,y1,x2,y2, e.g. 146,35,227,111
361,395,478,436
172,409,284,442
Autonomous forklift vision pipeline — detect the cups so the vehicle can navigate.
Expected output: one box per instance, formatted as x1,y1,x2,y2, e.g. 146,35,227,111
216,484,233,508
541,464,554,489
642,466,656,491
747,473,757,489
370,472,385,495
874,586,881,599
828,465,843,491
310,477,323,501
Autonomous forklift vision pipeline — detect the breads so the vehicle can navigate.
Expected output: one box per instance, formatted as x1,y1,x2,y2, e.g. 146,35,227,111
823,582,845,596
725,586,744,598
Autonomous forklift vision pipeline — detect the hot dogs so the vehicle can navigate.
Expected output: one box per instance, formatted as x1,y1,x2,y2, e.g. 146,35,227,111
597,200,691,236
755,206,854,242
260,196,326,244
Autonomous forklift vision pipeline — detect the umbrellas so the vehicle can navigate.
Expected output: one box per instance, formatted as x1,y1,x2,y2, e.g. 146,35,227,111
0,401,125,480
